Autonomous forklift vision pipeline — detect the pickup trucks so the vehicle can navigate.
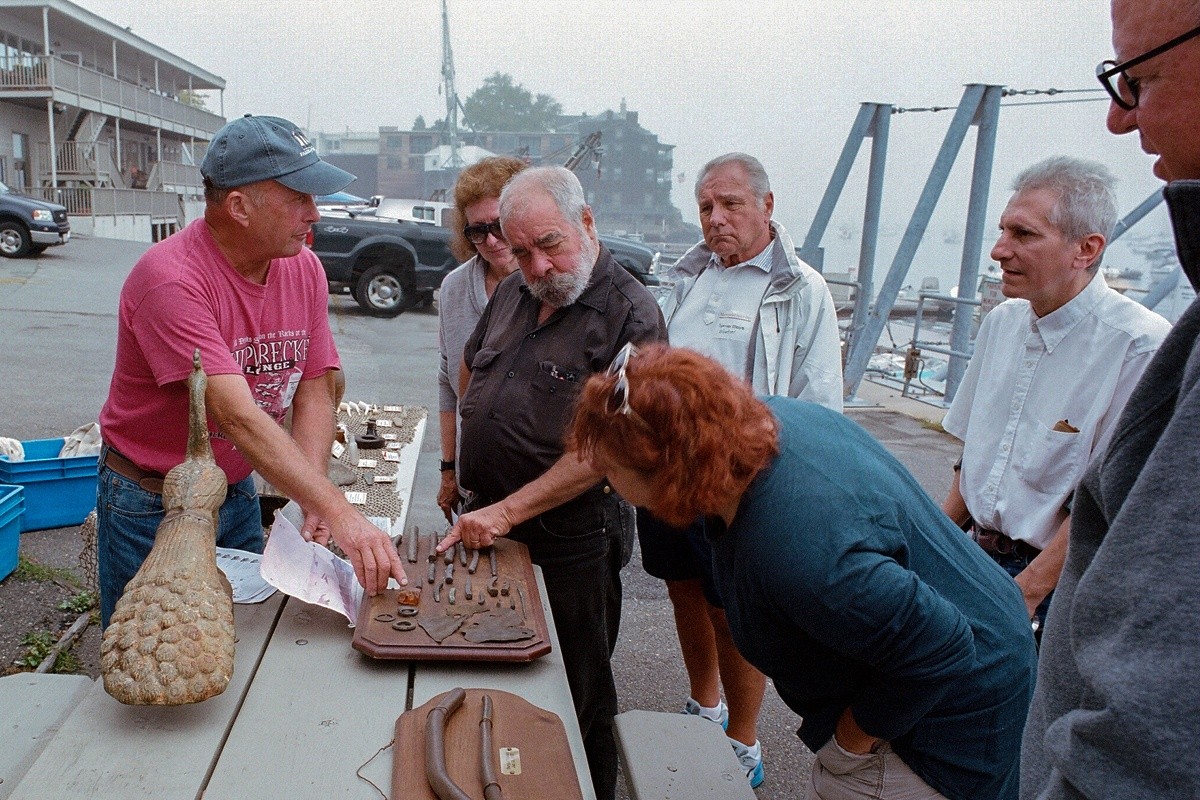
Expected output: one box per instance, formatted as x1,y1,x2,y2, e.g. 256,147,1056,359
305,210,662,319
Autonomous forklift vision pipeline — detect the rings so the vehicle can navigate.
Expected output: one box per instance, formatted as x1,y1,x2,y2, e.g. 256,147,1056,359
471,540,479,543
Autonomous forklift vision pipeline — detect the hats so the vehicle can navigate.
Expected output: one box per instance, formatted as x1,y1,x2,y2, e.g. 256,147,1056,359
199,112,358,197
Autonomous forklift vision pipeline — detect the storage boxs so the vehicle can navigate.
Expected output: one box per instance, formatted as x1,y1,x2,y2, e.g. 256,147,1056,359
0,484,26,585
0,438,100,533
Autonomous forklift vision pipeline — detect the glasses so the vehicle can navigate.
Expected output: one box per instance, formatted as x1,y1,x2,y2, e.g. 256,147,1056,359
1091,22,1200,110
462,217,507,245
602,342,657,439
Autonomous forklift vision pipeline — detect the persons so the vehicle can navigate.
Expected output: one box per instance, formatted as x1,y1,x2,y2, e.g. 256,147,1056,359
572,331,1035,800
432,154,529,527
636,150,844,786
436,164,672,800
95,112,410,635
1014,0,1198,800
940,155,1179,657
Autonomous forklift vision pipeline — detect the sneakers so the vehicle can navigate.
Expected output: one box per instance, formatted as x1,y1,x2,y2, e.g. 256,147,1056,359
678,696,731,735
726,737,767,789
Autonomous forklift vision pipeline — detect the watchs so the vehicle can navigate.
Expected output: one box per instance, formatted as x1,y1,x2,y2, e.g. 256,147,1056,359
440,459,456,471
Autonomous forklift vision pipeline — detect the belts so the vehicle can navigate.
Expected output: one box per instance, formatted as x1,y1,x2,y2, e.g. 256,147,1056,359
975,529,1012,555
99,443,166,496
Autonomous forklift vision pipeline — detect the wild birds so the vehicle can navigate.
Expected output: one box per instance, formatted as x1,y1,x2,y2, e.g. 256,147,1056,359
98,347,237,706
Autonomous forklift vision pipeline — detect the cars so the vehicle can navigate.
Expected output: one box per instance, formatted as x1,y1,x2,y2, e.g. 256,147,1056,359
0,179,72,260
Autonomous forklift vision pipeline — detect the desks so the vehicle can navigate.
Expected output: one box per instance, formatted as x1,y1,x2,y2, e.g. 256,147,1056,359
12,561,596,800
314,401,430,537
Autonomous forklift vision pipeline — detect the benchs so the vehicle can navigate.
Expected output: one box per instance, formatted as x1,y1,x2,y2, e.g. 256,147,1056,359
611,708,758,800
0,671,95,800
